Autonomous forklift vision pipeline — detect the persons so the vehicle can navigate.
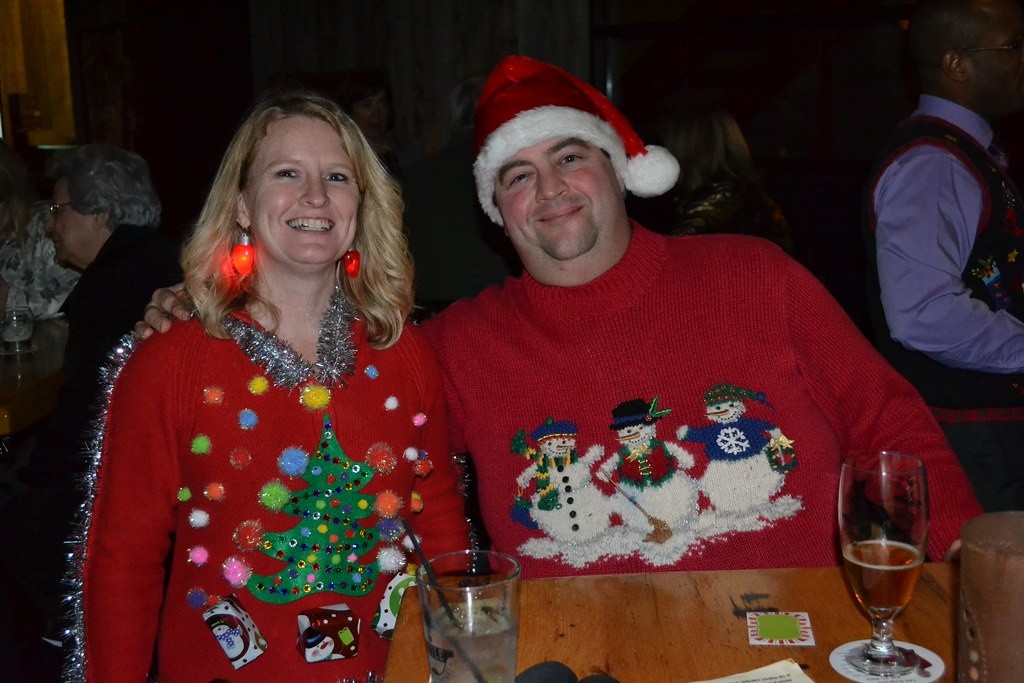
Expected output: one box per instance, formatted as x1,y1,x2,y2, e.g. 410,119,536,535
666,106,792,249
862,0,1024,512
1,145,179,590
138,57,986,580
347,75,424,176
0,143,90,321
67,95,480,683
397,75,520,326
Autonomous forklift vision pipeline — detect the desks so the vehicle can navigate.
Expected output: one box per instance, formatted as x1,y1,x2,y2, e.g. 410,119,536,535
381,561,958,683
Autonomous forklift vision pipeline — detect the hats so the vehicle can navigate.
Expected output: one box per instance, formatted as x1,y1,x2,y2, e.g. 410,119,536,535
473,55,682,227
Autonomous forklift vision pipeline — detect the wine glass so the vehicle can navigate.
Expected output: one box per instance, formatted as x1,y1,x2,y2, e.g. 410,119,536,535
837,450,929,676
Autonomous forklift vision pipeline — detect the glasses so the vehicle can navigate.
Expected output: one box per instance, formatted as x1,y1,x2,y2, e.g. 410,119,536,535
49,202,73,220
941,35,1024,62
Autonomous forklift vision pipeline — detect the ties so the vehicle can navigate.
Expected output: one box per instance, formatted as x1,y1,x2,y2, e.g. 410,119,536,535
991,141,1014,184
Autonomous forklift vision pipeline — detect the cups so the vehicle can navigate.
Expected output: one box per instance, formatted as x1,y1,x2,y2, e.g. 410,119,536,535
416,549,521,682
0,305,34,354
